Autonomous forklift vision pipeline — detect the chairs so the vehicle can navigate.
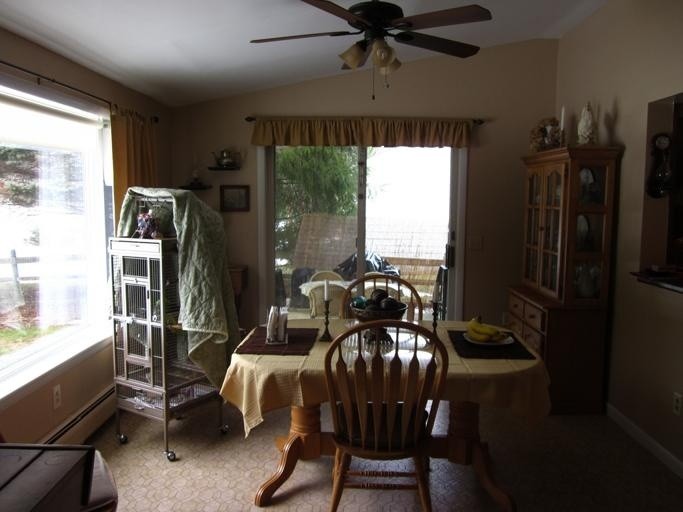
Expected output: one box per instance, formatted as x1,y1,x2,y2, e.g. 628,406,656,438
307,270,447,511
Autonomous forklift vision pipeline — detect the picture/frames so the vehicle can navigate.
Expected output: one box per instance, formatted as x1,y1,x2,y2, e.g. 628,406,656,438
219,184,250,214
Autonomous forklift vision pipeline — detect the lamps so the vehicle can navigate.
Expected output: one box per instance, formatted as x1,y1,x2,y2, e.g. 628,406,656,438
335,27,403,101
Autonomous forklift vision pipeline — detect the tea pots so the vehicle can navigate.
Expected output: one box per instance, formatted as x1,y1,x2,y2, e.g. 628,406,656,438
210,149,242,168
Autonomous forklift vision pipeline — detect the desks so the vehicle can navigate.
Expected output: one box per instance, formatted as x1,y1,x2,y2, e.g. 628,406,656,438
228,320,544,511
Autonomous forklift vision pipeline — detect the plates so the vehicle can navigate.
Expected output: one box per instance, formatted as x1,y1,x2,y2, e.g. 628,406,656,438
461,332,515,346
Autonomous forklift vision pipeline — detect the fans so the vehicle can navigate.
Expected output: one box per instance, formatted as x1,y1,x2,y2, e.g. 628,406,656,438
248,0,492,71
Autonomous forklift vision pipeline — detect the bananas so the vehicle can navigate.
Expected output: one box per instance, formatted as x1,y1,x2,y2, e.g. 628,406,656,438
466,314,502,343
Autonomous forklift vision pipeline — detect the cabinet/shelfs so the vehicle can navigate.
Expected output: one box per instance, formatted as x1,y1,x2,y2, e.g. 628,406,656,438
108,236,224,420
502,147,624,416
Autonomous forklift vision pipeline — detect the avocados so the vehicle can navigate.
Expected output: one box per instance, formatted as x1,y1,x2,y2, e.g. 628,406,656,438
350,288,398,311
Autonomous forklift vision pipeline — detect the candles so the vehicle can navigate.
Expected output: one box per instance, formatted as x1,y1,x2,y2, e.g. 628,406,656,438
561,104,566,130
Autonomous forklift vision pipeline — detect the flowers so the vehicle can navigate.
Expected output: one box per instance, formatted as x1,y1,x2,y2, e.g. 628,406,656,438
531,115,560,148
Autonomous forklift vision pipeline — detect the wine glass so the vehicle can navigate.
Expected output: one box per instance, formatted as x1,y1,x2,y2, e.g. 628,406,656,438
349,299,410,345
